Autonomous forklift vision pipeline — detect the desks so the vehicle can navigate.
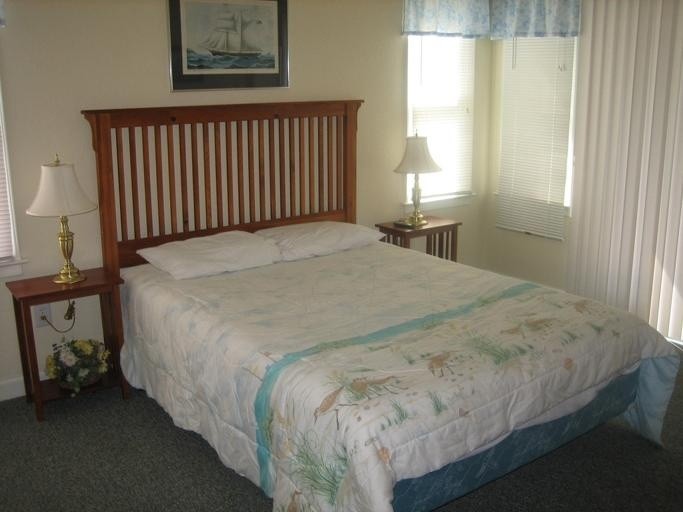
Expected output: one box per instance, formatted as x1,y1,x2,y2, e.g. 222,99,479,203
374,215,462,263
4,267,133,422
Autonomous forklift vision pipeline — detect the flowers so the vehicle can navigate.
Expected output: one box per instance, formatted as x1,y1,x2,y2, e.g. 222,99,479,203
40,329,117,397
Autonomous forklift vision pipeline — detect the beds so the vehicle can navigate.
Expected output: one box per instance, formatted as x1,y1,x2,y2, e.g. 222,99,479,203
79,100,642,510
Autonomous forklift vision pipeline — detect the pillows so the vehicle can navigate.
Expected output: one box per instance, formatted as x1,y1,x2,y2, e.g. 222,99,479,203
134,220,386,280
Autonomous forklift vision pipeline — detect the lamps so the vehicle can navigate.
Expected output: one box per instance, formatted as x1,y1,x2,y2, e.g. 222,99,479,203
393,127,445,227
22,151,99,286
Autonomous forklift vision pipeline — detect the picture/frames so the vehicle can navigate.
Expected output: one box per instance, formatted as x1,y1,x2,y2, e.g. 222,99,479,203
168,1,290,95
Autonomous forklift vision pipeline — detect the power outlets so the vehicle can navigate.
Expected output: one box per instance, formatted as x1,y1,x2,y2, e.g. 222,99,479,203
31,303,52,329
